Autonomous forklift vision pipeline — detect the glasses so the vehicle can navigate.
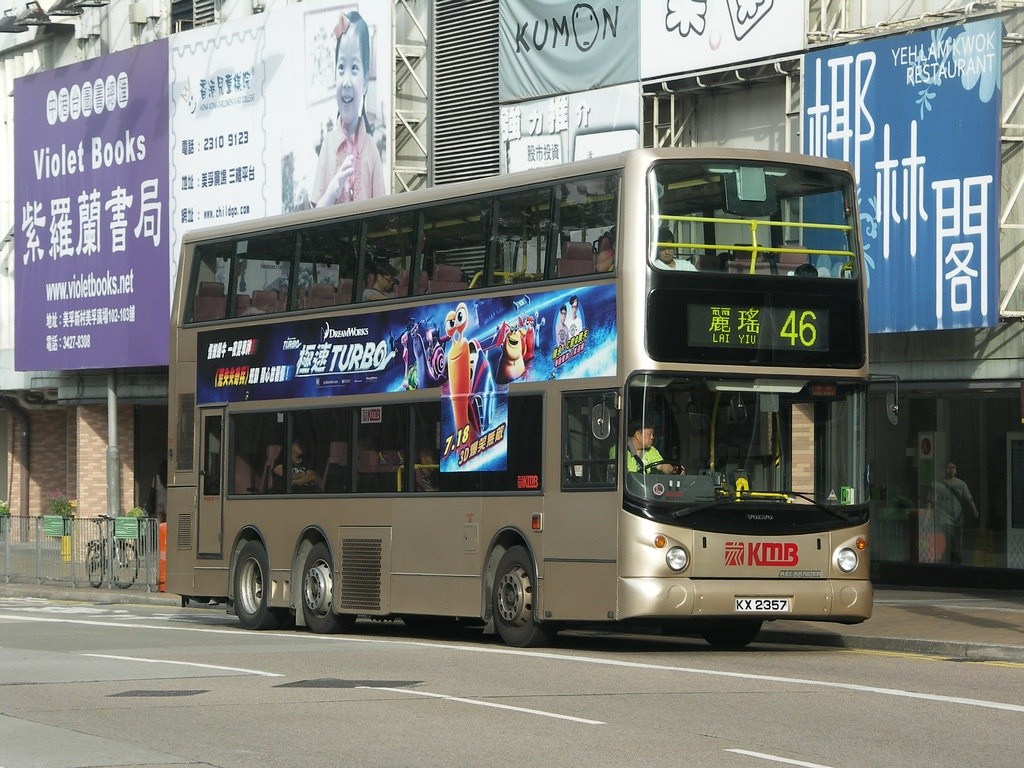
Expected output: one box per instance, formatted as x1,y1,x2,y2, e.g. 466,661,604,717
383,276,392,283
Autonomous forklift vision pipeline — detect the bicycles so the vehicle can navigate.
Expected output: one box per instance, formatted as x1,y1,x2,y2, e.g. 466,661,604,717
84,514,139,589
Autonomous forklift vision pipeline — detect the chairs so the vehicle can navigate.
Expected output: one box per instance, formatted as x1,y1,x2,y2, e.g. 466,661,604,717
192,277,353,323
322,443,350,494
416,452,441,492
692,244,812,277
557,234,612,279
355,449,404,491
260,445,283,491
395,264,469,297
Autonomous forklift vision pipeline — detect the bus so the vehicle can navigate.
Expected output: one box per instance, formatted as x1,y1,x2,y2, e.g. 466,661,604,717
165,144,901,648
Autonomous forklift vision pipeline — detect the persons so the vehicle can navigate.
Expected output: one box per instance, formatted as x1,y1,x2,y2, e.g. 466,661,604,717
654,228,698,271
152,460,166,522
360,261,398,302
415,447,447,491
787,264,819,277
270,431,324,494
567,295,583,341
556,304,569,348
304,9,388,210
606,417,687,485
921,460,979,564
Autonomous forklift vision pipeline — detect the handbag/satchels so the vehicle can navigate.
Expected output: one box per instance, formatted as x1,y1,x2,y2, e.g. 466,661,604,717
962,502,974,520
146,475,157,514
